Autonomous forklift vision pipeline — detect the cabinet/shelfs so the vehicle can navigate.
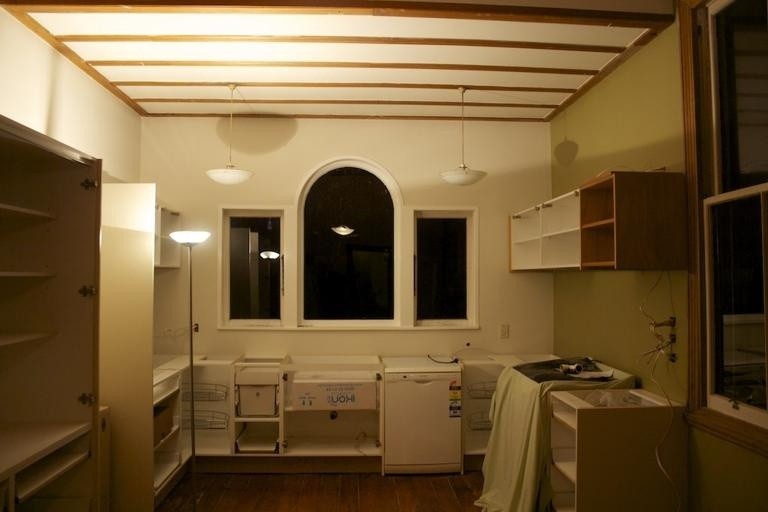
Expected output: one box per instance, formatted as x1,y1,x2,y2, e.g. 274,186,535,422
232,353,287,472
549,389,690,512
508,172,686,270
0,113,103,512
101,179,182,512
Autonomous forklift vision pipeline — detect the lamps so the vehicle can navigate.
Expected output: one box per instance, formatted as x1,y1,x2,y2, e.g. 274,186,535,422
259,250,279,319
439,86,487,186
168,232,209,510
206,84,254,186
331,194,354,236
